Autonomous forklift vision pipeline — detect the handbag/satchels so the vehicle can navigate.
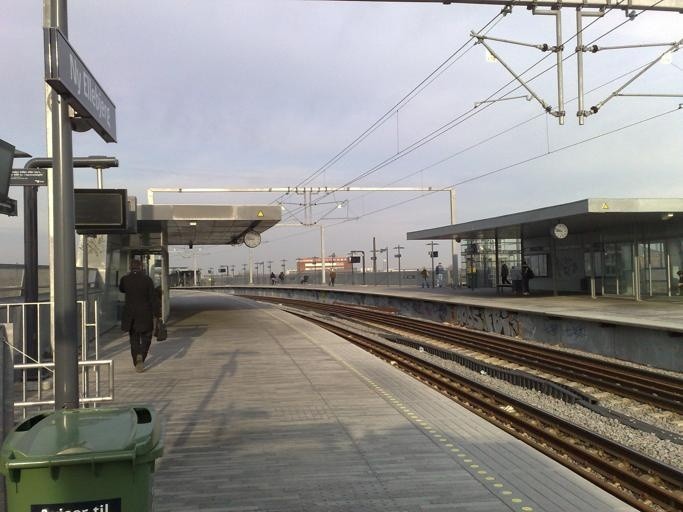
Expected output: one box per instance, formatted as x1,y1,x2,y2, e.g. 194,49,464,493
154,317,167,341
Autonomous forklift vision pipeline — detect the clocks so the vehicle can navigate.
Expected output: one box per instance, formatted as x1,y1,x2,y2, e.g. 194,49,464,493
550,223,568,239
244,230,261,248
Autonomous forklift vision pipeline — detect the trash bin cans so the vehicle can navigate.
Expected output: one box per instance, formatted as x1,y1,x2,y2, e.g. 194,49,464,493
0,405,163,512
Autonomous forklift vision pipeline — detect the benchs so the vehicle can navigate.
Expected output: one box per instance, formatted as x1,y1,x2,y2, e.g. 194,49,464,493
496,285,522,296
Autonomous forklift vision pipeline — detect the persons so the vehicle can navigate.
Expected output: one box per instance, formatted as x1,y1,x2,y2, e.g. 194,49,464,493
280,272,285,284
435,263,444,287
520,261,530,296
329,268,336,287
119,260,160,372
421,267,429,288
270,272,276,285
501,261,511,284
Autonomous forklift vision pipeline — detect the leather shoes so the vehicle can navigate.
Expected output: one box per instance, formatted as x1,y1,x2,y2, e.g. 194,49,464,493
135,354,144,373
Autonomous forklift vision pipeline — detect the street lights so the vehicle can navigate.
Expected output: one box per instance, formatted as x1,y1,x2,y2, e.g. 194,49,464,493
392,245,404,285
379,246,389,285
426,241,438,288
209,251,365,286
